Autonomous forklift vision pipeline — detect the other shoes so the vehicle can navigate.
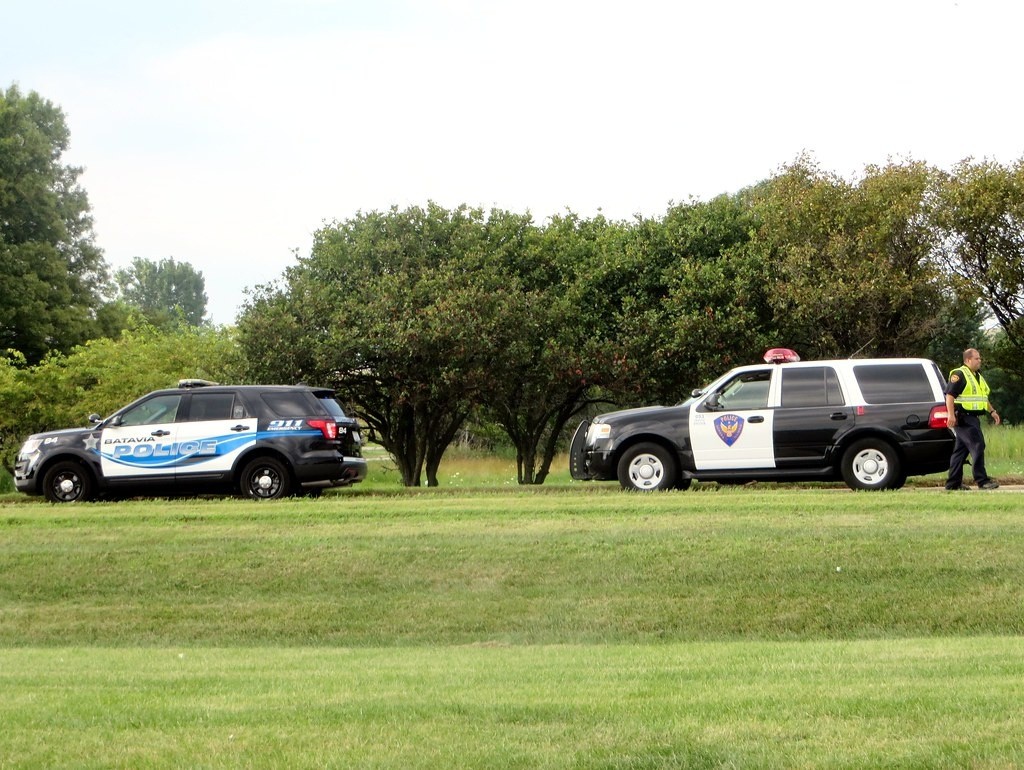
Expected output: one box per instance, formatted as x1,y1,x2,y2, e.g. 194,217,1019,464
948,484,971,490
979,483,1000,491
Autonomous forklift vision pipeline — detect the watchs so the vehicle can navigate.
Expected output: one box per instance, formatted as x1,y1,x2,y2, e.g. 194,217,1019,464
990,410,997,415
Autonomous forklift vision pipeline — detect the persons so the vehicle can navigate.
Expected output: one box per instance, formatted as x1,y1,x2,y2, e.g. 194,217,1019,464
944,348,1001,491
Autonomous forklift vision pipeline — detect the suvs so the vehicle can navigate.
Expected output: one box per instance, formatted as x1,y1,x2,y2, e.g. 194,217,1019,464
15,379,368,503
568,348,972,491
